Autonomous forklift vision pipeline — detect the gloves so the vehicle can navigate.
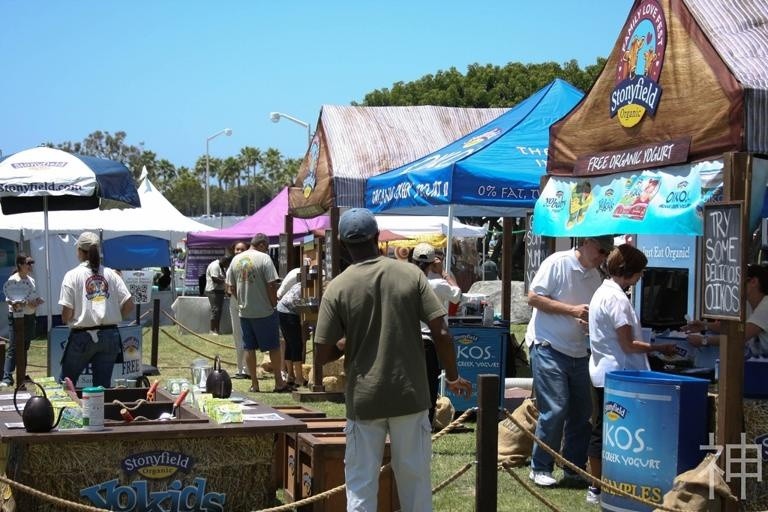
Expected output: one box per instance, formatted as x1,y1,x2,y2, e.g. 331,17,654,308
445,377,473,399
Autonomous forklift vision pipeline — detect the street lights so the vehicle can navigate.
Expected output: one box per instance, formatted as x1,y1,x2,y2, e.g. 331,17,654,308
204,127,232,219
269,111,315,153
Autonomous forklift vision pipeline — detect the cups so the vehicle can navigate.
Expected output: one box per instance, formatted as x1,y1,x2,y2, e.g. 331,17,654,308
168,378,189,394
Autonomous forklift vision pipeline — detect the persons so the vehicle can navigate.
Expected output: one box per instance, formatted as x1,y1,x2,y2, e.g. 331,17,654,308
58,232,135,388
680,264,768,360
0,253,46,387
154,266,171,291
585,244,679,503
408,243,498,426
204,233,308,394
524,235,614,486
315,208,472,512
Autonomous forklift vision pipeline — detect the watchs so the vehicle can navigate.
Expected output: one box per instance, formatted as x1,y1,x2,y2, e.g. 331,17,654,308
701,335,710,347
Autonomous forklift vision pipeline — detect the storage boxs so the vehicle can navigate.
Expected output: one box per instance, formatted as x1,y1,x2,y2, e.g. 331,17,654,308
270,405,400,511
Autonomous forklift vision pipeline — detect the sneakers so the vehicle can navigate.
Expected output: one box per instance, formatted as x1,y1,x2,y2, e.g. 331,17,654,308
585,485,603,504
528,468,557,487
235,366,308,392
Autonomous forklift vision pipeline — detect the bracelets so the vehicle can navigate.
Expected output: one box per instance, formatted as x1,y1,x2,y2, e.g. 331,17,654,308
703,319,707,331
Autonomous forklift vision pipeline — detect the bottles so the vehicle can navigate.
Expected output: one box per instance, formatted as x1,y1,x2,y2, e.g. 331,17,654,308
114,378,126,388
483,295,494,328
126,379,138,388
191,357,213,392
82,386,106,430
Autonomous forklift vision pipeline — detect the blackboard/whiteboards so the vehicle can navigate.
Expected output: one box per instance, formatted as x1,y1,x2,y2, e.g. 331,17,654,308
524,215,555,296
701,200,745,323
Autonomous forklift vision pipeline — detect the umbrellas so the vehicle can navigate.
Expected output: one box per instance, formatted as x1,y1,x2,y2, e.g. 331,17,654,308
0,141,141,376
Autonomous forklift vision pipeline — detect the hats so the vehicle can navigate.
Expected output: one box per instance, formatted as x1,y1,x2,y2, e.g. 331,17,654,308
73,231,101,251
338,207,379,244
412,242,436,265
596,235,616,252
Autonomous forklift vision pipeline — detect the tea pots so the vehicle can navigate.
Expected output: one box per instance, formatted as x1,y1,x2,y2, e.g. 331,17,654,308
13,380,67,433
205,353,232,399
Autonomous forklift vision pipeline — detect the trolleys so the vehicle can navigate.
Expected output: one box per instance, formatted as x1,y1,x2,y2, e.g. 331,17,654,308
46,321,152,400
